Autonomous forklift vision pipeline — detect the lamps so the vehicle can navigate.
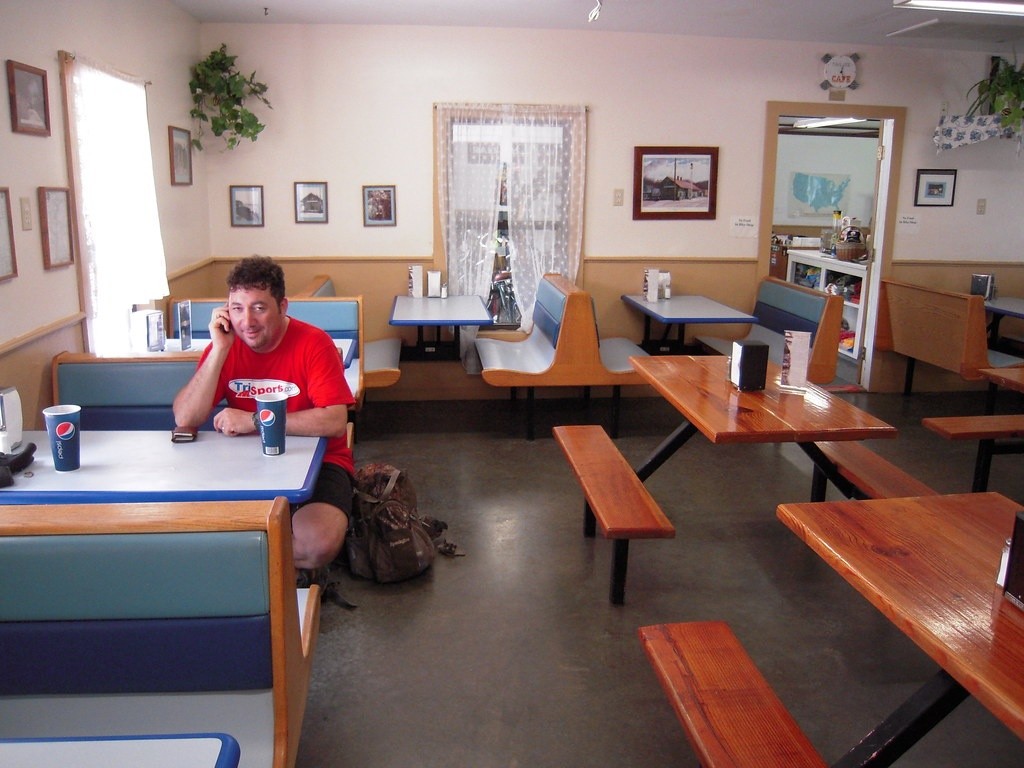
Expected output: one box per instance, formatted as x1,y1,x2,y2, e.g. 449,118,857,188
892,0,1024,17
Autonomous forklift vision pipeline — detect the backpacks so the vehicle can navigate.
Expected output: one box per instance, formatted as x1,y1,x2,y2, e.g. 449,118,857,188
341,463,435,586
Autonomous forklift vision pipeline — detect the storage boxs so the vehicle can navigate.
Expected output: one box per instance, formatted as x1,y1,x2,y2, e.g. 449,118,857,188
730,339,770,391
659,271,672,299
427,270,441,297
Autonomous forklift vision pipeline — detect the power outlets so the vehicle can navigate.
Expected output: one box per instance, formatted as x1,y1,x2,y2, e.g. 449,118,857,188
20,197,33,231
613,189,624,206
939,100,949,116
976,198,986,215
660,347,670,352
425,347,436,352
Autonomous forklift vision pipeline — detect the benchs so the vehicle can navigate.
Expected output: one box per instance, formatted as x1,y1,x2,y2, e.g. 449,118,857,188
637,617,834,768
922,415,1024,492
809,442,944,503
693,275,844,384
473,272,654,436
0,275,402,768
873,278,1024,416
552,424,676,607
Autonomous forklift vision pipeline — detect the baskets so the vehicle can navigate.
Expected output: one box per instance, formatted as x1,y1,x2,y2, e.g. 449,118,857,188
836,226,866,260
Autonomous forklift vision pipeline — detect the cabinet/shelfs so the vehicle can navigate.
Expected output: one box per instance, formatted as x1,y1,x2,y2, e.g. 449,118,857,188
785,248,868,359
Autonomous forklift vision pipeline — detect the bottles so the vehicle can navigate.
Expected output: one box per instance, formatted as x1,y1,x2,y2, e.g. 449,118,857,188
441,284,447,299
831,233,837,256
0,425,12,454
665,285,671,299
725,355,732,382
995,538,1012,590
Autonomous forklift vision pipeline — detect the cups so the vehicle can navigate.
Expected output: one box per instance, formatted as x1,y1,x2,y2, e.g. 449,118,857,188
254,392,289,457
42,404,81,474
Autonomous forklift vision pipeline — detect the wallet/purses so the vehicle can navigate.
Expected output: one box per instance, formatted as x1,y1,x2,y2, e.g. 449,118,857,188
172,426,197,442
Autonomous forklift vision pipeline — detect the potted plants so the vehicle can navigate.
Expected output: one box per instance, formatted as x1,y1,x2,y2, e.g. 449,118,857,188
964,46,1024,133
188,42,275,155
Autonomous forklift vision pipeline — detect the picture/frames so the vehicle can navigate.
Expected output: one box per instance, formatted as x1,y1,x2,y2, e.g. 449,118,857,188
914,168,957,206
0,186,19,281
229,185,264,228
632,146,719,222
37,186,75,270
168,125,195,186
6,59,52,138
362,184,397,227
294,181,329,224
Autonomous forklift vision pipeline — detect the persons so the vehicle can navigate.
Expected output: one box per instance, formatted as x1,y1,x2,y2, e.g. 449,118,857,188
173,255,357,570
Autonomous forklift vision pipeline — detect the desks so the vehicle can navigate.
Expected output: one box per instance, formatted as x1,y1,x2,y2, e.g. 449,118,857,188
163,336,358,369
971,366,1024,491
0,429,328,504
623,292,758,356
389,294,493,364
982,296,1024,359
627,349,899,483
776,487,1024,742
0,733,240,768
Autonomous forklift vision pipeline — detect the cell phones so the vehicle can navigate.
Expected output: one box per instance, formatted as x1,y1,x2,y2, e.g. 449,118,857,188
220,316,232,333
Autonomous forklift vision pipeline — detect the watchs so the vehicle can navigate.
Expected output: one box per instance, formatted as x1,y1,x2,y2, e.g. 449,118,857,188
252,412,261,431
